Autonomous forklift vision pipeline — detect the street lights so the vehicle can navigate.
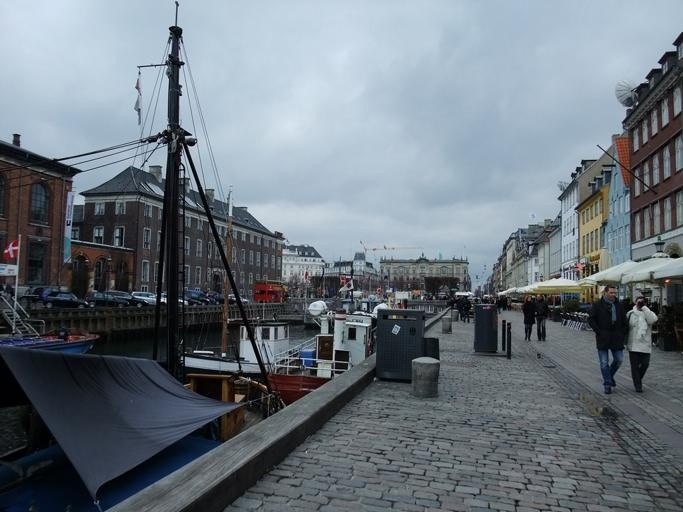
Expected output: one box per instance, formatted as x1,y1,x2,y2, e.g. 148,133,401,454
651,234,668,314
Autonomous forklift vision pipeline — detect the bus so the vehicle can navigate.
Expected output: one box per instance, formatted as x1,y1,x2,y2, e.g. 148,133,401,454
252,284,284,302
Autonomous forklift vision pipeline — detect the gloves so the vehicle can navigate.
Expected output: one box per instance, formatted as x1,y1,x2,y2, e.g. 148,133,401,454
637,301,645,308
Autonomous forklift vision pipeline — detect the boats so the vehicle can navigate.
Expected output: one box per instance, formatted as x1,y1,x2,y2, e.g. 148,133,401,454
265,310,377,406
0,374,285,512
0,329,100,354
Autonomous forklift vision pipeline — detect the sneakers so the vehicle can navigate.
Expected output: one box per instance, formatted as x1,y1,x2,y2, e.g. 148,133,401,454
604,377,616,393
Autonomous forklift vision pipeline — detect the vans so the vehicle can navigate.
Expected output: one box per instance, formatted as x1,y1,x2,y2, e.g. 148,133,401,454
0,284,248,309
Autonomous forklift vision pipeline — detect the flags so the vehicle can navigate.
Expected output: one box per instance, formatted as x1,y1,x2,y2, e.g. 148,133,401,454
337,278,354,293
3,237,18,262
382,271,387,282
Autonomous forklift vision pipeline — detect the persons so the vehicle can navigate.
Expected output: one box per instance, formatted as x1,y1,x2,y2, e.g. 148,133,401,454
411,291,563,327
519,294,535,342
625,295,658,394
619,295,682,349
586,283,626,395
532,293,552,341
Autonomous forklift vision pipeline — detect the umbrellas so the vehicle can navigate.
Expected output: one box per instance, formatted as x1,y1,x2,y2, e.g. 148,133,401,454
494,276,580,307
619,250,670,281
579,257,643,300
622,256,682,291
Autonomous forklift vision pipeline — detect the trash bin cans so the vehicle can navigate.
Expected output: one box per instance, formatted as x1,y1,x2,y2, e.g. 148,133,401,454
374,309,426,384
473,304,498,353
